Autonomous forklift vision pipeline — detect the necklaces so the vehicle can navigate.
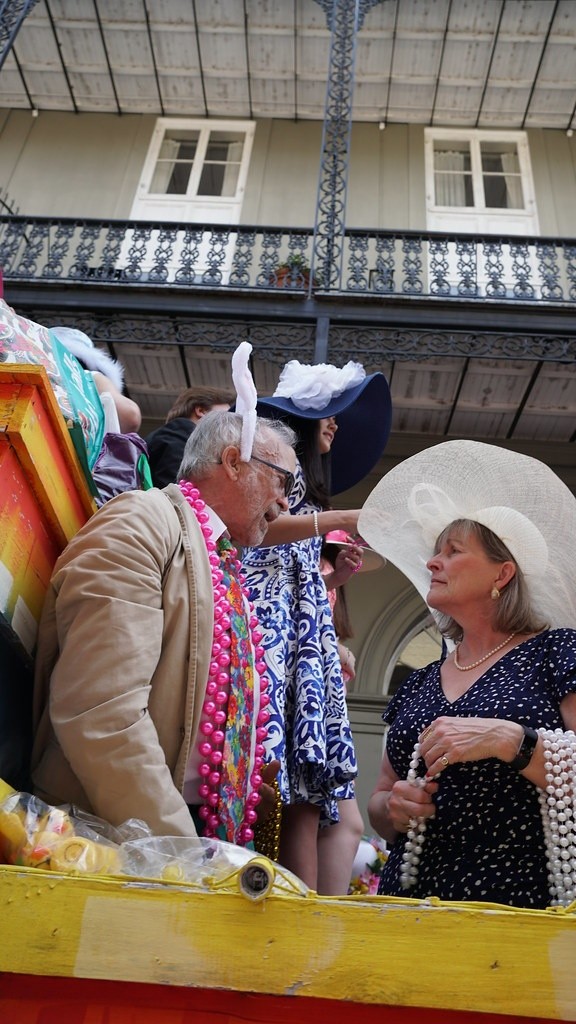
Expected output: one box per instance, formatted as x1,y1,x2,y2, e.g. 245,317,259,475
454,633,514,671
177,478,268,844
536,727,576,907
255,762,280,863
399,733,441,888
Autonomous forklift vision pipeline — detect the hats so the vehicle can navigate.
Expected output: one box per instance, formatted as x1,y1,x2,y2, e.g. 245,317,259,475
323,529,386,574
48,327,124,395
227,357,393,498
357,440,576,628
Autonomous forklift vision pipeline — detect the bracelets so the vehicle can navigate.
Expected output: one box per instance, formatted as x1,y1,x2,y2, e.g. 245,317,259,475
509,724,538,773
314,511,319,537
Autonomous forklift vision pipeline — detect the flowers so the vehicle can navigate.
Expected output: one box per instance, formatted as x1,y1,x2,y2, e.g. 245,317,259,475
271,360,366,412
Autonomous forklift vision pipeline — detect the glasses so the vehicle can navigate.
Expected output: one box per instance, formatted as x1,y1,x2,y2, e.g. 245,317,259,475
252,452,296,497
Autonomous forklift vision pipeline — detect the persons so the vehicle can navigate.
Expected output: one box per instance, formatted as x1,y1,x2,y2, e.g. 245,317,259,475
43,323,393,897
366,506,576,910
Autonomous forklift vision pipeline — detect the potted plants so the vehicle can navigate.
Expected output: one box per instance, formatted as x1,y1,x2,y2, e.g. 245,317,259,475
270,253,327,289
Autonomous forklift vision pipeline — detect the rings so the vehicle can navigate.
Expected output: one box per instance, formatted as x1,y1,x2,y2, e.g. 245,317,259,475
441,756,449,767
